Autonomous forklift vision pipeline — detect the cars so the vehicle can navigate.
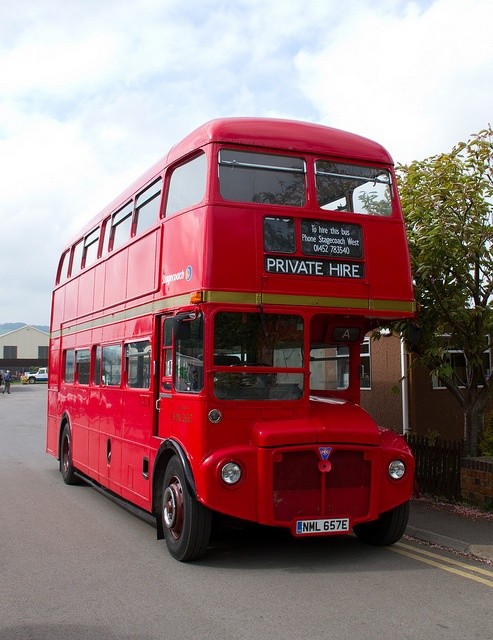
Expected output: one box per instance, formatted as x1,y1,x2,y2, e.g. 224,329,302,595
25,368,48,384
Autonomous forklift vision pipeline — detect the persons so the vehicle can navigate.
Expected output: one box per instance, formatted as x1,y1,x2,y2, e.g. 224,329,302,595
1,370,11,394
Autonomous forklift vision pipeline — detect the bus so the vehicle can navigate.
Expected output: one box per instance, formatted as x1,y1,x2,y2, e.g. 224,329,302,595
44,116,413,563
